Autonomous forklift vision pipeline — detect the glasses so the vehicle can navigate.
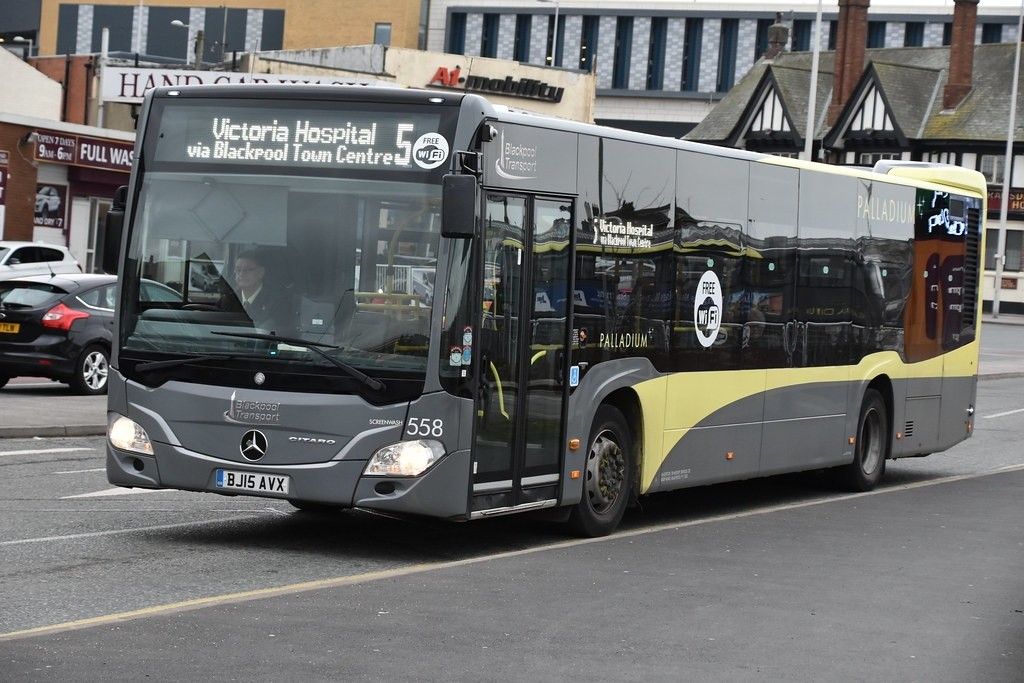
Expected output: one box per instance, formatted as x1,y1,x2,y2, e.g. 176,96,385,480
233,268,260,273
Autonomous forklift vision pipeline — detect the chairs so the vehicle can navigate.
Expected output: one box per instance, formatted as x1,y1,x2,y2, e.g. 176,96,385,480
347,310,393,353
616,275,779,354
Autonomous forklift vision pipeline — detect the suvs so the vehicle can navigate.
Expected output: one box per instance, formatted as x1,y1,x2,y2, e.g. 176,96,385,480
0,274,192,396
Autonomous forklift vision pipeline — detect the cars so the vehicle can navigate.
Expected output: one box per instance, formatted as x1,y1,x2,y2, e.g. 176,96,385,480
593,258,657,294
0,240,82,280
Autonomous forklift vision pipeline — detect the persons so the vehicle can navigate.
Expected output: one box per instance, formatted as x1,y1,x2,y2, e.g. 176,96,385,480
227,250,285,330
749,310,765,354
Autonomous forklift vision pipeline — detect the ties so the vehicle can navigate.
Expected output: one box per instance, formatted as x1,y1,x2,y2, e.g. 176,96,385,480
244,301,249,311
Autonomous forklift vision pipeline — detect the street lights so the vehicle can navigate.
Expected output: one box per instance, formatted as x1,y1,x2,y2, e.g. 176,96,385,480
171,19,206,69
14,36,34,55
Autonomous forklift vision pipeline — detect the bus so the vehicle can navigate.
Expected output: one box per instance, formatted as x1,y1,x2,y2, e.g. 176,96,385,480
105,85,990,539
739,244,904,343
